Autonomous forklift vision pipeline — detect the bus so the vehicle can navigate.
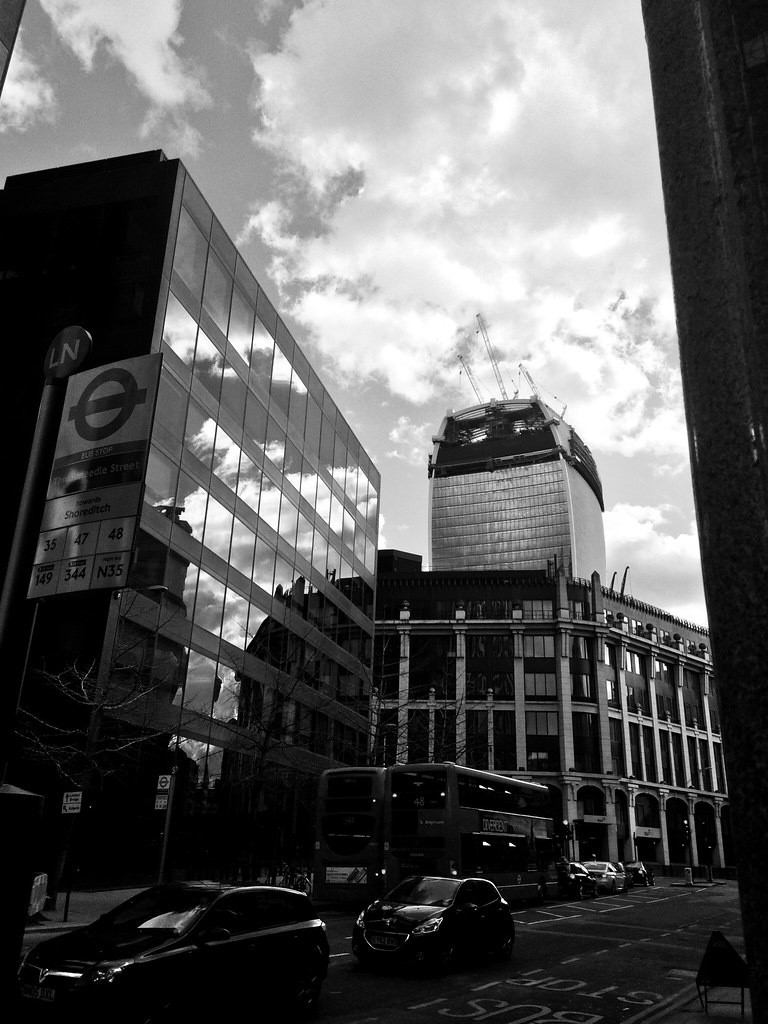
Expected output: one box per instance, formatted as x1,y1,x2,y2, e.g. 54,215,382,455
312,766,397,904
382,760,566,905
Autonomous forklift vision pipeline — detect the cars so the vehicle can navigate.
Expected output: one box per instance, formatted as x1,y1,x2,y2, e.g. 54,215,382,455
623,860,656,887
583,861,623,895
612,861,636,889
349,877,516,974
565,861,602,901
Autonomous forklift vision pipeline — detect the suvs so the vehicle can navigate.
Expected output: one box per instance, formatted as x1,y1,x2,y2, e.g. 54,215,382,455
16,881,332,1024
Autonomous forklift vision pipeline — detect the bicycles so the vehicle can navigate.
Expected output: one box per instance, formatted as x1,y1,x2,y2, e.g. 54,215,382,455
279,865,312,898
